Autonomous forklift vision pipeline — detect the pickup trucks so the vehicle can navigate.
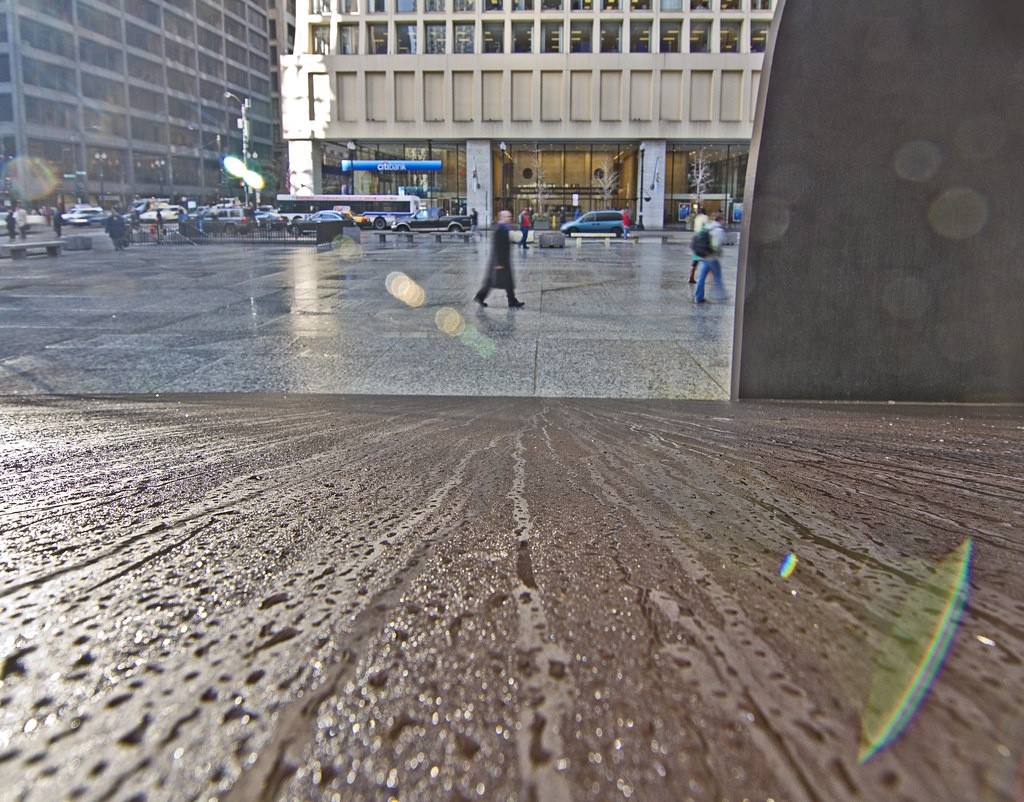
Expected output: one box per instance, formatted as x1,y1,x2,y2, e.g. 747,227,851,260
390,207,474,236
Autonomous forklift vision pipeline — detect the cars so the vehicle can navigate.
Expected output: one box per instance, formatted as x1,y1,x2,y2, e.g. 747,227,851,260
0,196,279,238
292,211,356,239
557,210,626,237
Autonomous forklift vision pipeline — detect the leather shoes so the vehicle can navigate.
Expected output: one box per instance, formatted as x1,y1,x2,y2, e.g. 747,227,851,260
508,298,526,307
473,295,488,306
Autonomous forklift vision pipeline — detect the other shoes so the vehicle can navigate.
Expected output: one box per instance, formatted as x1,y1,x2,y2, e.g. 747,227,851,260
688,280,697,284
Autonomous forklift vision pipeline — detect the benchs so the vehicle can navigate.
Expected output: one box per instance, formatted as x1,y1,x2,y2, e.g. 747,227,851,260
571,231,617,249
429,231,474,244
1,240,68,260
373,230,419,243
628,232,675,246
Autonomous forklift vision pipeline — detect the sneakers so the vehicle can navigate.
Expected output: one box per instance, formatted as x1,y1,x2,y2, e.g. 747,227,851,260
695,298,706,303
715,297,732,302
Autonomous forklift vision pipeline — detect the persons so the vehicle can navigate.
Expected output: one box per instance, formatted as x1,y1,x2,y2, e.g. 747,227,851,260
155,209,163,230
52,209,64,238
621,205,631,238
694,214,733,306
5,209,18,242
130,208,140,234
685,207,712,287
14,205,29,241
470,207,525,308
517,206,529,250
104,209,126,250
469,207,483,239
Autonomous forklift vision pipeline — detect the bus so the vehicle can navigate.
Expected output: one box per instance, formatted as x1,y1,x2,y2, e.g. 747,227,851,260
275,193,421,231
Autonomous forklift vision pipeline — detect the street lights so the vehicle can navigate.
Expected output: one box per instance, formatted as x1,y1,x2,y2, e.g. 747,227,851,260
635,141,647,230
346,140,359,193
499,140,508,214
222,91,253,208
